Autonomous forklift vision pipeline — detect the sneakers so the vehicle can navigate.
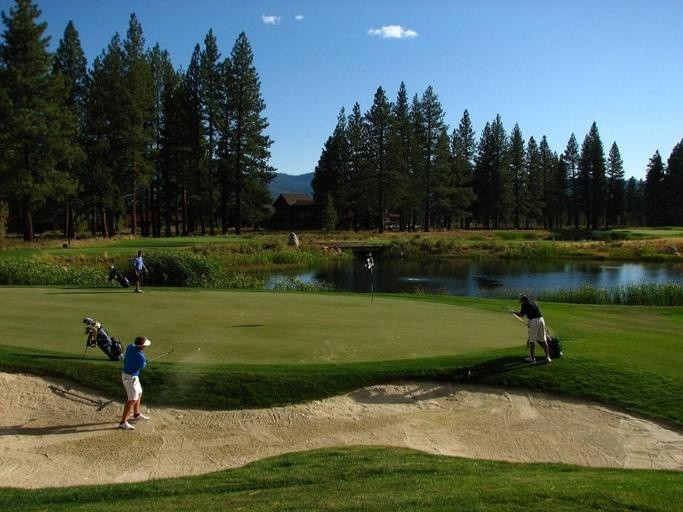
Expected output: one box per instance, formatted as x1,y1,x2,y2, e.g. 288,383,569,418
132,412,151,421
135,288,143,293
119,420,135,430
524,357,536,362
543,355,551,363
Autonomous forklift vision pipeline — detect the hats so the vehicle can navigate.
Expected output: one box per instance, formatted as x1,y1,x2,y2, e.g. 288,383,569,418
517,294,526,304
133,337,151,347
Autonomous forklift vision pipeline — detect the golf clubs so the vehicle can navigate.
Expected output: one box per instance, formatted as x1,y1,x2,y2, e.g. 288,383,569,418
146,347,174,363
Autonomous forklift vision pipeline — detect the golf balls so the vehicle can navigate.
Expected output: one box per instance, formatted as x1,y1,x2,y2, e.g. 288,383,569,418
197,348,200,350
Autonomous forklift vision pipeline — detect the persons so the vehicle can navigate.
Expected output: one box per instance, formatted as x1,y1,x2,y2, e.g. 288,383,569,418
511,294,552,364
119,336,151,431
133,251,148,293
